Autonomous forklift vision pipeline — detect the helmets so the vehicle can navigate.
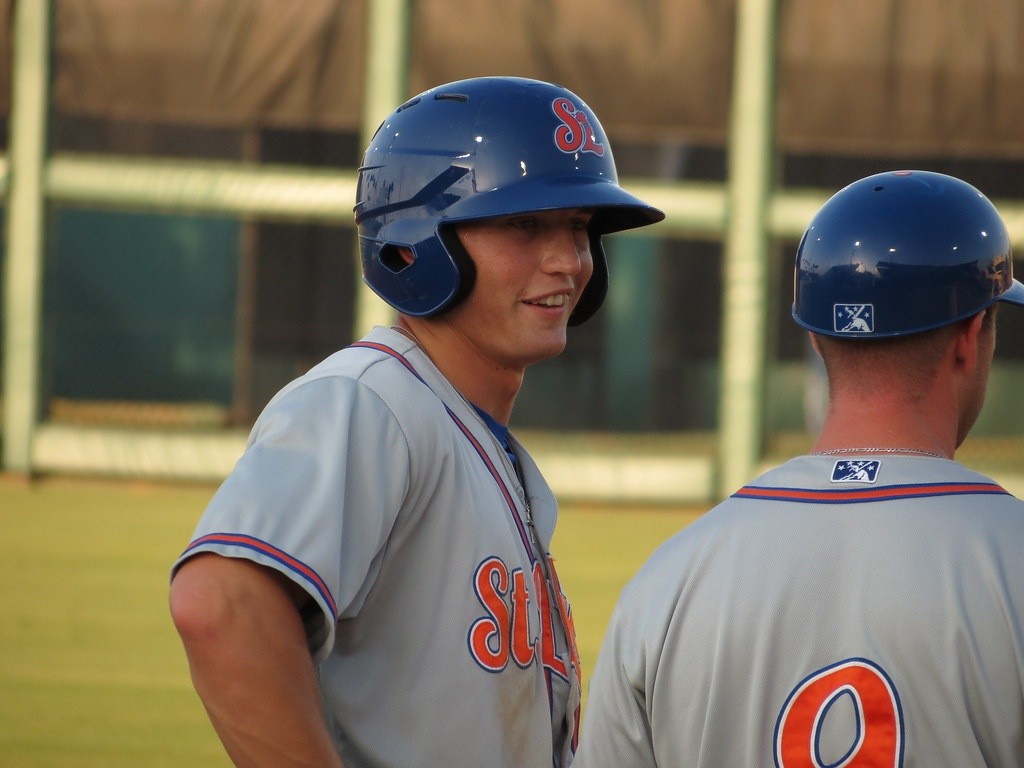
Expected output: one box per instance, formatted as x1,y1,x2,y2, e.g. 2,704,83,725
791,169,1024,339
354,76,665,327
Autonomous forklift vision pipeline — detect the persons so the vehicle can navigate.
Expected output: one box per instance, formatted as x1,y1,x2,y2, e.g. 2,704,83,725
561,166,1024,768
163,74,669,767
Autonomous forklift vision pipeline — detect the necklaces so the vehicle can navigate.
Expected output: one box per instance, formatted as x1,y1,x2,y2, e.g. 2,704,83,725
388,324,536,548
806,447,942,459
